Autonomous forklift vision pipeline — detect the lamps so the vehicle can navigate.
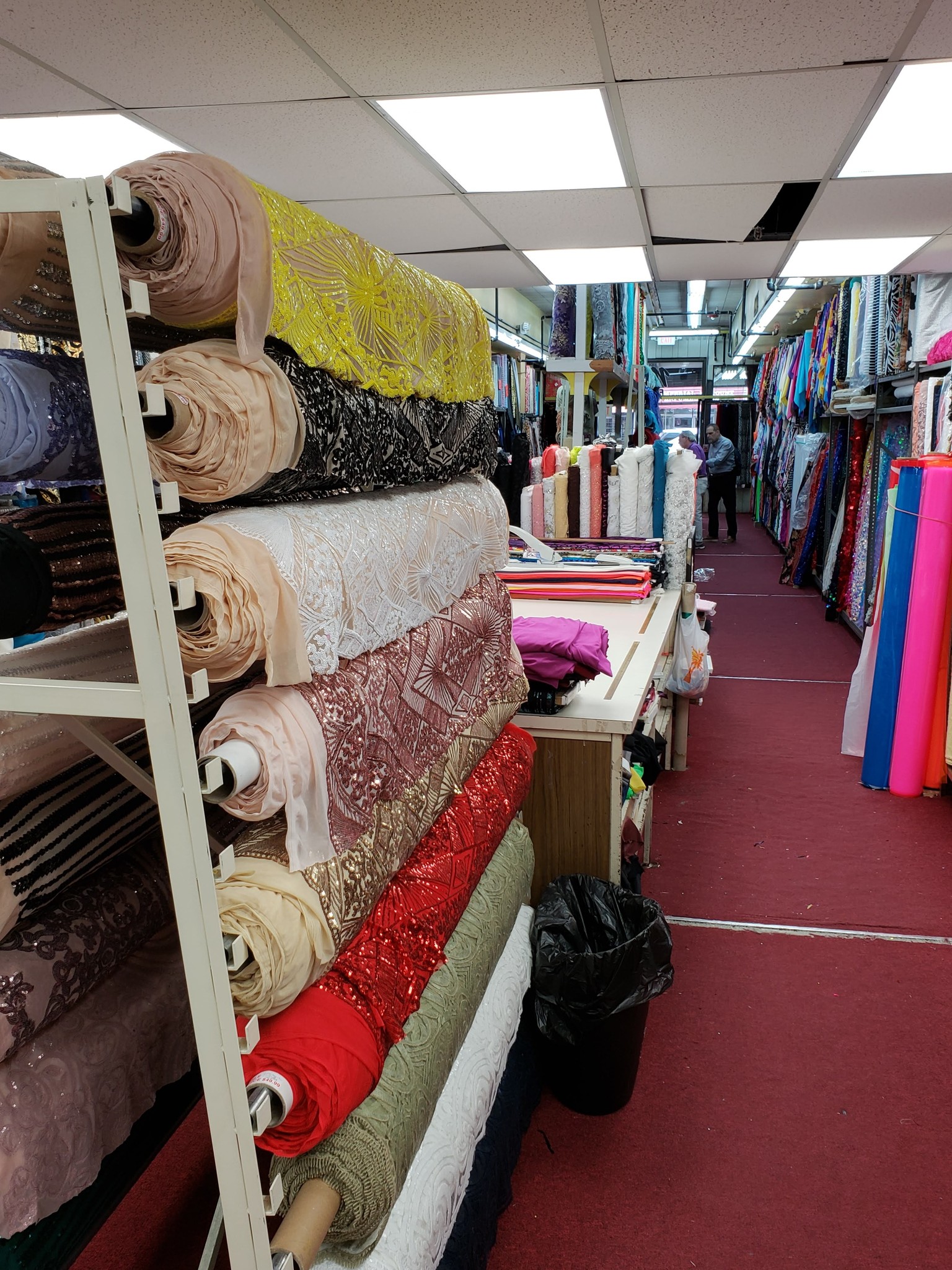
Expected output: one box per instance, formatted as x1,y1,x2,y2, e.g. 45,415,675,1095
648,277,806,380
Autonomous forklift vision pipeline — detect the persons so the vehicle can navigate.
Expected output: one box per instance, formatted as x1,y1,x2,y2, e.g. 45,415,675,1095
705,424,737,543
678,431,708,548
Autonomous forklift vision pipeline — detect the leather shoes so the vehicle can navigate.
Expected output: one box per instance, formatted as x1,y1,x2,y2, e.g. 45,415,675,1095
702,537,718,541
722,535,736,543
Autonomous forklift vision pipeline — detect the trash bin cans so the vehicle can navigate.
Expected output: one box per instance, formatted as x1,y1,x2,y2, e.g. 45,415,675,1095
532,875,673,1117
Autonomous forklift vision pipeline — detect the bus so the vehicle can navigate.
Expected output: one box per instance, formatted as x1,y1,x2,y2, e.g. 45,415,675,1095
657,404,717,442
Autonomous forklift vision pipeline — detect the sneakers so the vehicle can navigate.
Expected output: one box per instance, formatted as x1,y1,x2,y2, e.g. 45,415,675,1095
695,542,705,549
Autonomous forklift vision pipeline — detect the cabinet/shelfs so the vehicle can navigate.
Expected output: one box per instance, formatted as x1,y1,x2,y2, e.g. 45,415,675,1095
511,587,683,909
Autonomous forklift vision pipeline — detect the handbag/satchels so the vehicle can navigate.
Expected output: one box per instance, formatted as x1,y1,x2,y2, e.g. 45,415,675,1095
665,594,709,697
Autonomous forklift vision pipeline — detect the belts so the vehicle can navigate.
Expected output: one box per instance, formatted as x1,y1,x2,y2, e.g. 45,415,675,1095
696,475,707,479
